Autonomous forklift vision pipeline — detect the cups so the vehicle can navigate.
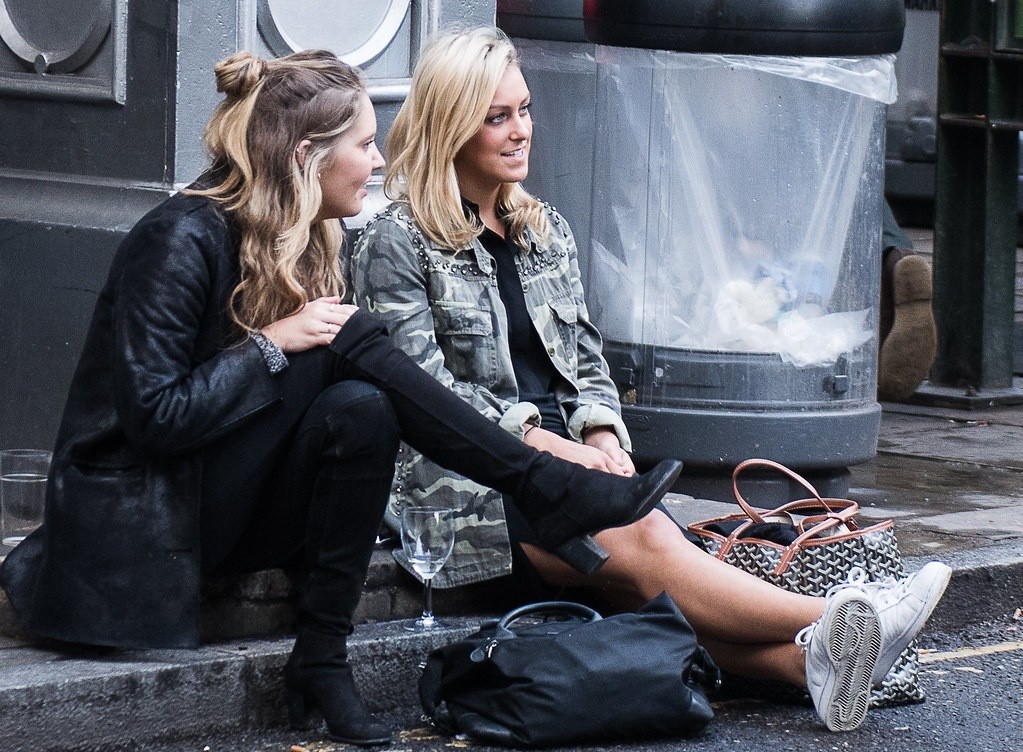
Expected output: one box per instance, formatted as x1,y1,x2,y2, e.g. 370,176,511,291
0,448,54,562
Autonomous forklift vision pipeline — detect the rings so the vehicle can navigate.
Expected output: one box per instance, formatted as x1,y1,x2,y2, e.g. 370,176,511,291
329,303,335,312
328,323,332,332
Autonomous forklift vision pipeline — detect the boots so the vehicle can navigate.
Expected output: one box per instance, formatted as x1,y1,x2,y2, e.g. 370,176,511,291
281,390,401,744
324,306,685,578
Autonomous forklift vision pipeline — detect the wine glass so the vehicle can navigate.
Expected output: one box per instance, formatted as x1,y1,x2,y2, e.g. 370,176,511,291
400,506,455,633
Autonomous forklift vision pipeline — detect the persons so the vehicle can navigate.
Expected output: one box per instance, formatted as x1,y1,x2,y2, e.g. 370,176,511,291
348,21,952,733
0,49,686,747
877,196,938,400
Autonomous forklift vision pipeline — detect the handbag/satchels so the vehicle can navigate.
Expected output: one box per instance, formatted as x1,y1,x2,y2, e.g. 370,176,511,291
411,589,721,750
688,459,927,708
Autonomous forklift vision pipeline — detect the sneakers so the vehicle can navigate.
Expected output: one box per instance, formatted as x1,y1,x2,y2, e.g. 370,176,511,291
795,587,884,732
824,562,955,690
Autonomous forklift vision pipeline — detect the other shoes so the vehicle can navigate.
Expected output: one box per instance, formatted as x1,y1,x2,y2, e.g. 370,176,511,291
878,252,936,402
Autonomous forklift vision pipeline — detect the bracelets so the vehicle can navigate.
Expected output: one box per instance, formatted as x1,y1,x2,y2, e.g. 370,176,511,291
524,425,536,436
